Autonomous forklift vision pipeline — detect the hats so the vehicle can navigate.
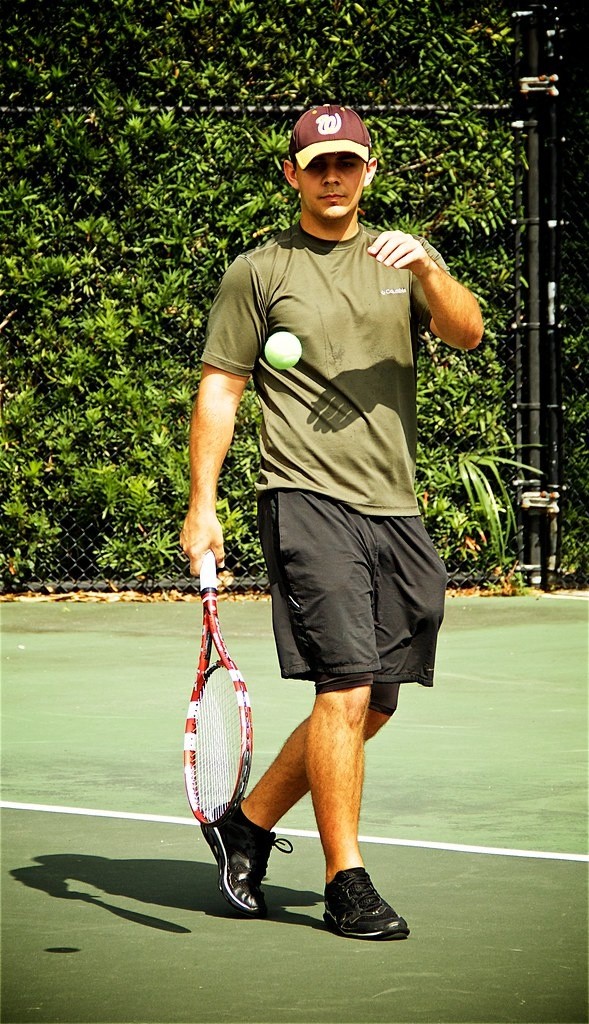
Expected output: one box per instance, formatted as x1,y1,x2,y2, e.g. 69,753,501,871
288,104,372,170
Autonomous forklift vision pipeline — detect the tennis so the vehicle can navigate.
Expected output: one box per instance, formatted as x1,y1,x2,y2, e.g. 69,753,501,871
264,330,305,372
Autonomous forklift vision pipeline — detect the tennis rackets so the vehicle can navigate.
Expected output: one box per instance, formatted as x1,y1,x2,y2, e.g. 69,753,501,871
182,547,253,828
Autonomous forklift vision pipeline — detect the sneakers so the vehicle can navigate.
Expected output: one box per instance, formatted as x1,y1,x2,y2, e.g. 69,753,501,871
323,867,410,940
200,797,293,917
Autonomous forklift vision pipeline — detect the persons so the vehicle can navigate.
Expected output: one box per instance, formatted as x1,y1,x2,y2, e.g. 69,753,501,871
176,106,488,938
